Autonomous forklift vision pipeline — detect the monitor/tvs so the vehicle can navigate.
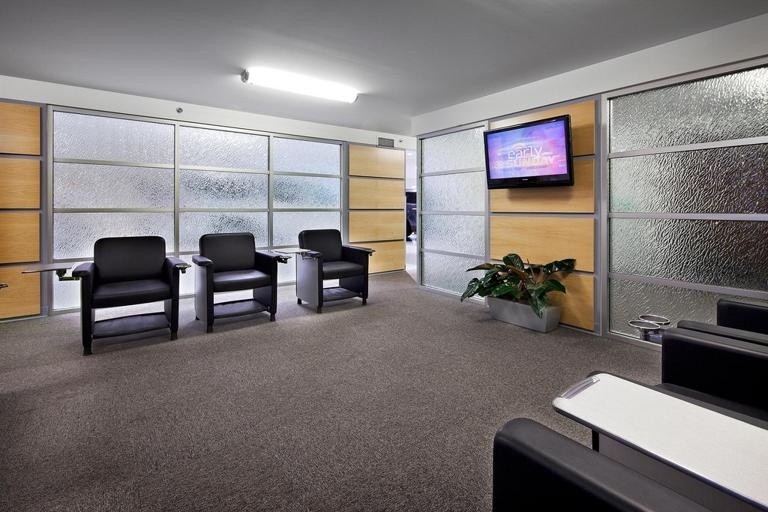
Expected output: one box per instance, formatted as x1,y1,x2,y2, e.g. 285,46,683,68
483,114,574,189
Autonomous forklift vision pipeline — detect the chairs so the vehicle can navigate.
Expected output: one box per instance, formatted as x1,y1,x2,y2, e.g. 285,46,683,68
191,232,292,335
294,230,377,313
71,230,192,357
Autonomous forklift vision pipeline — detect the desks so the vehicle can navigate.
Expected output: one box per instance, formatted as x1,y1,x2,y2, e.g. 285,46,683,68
275,247,311,255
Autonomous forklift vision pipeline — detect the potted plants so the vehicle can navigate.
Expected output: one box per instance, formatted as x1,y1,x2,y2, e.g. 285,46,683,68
458,252,577,334
